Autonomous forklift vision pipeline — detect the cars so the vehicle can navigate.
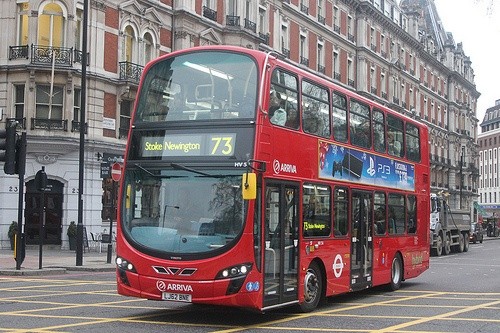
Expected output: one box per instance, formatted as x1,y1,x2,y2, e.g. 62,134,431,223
469,222,482,243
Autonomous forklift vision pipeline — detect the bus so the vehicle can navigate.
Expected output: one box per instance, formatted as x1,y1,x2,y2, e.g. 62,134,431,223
115,46,429,315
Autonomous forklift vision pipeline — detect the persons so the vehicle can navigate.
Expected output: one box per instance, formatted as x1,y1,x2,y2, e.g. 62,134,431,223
168,188,201,234
260,198,413,269
268,91,287,127
158,93,189,121
482,221,493,237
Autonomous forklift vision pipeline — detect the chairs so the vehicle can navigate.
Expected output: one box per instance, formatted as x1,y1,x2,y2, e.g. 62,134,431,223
89,229,115,254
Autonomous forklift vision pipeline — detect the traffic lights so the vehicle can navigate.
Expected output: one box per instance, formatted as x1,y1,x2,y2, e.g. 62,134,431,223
0,118,26,175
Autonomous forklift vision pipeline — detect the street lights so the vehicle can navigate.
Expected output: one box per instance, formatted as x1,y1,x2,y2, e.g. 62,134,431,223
460,155,480,209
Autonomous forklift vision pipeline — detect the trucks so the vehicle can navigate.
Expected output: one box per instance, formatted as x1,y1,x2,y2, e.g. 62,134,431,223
430,193,471,257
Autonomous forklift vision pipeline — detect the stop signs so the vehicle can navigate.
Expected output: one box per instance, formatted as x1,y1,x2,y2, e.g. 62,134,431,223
112,163,121,182
